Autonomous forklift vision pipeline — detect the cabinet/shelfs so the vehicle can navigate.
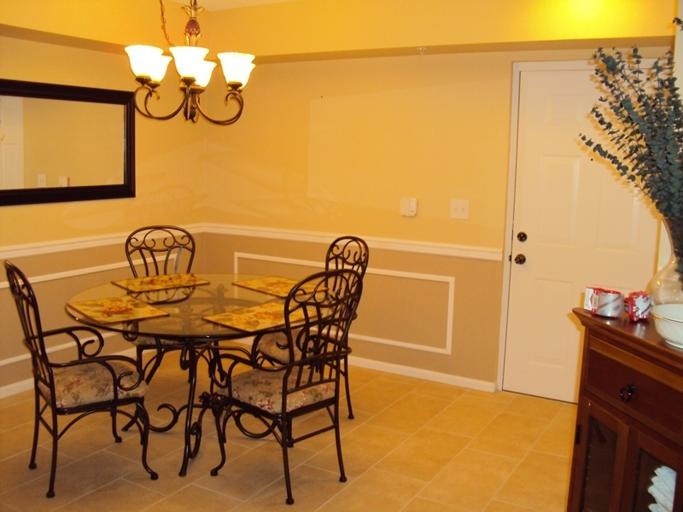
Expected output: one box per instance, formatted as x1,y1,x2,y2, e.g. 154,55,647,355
567,307,683,512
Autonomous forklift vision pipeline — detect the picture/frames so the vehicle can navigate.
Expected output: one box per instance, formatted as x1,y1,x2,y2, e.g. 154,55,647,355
0,80,136,205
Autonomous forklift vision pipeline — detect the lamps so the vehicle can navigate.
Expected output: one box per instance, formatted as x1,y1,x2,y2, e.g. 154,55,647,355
124,0,258,125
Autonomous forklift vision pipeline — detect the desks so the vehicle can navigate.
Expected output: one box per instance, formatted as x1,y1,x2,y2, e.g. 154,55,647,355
64,271,346,480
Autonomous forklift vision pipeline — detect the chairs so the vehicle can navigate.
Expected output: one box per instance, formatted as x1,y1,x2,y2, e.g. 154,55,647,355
3,259,160,500
256,235,369,419
178,268,368,505
121,223,209,386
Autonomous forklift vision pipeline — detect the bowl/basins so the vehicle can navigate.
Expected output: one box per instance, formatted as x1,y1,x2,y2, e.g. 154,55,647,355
650,303,683,349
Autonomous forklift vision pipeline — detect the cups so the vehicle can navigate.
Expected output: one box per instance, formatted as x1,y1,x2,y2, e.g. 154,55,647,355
596,292,624,318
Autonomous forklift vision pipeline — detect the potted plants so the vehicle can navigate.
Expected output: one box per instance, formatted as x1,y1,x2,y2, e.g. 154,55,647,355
578,17,683,306
